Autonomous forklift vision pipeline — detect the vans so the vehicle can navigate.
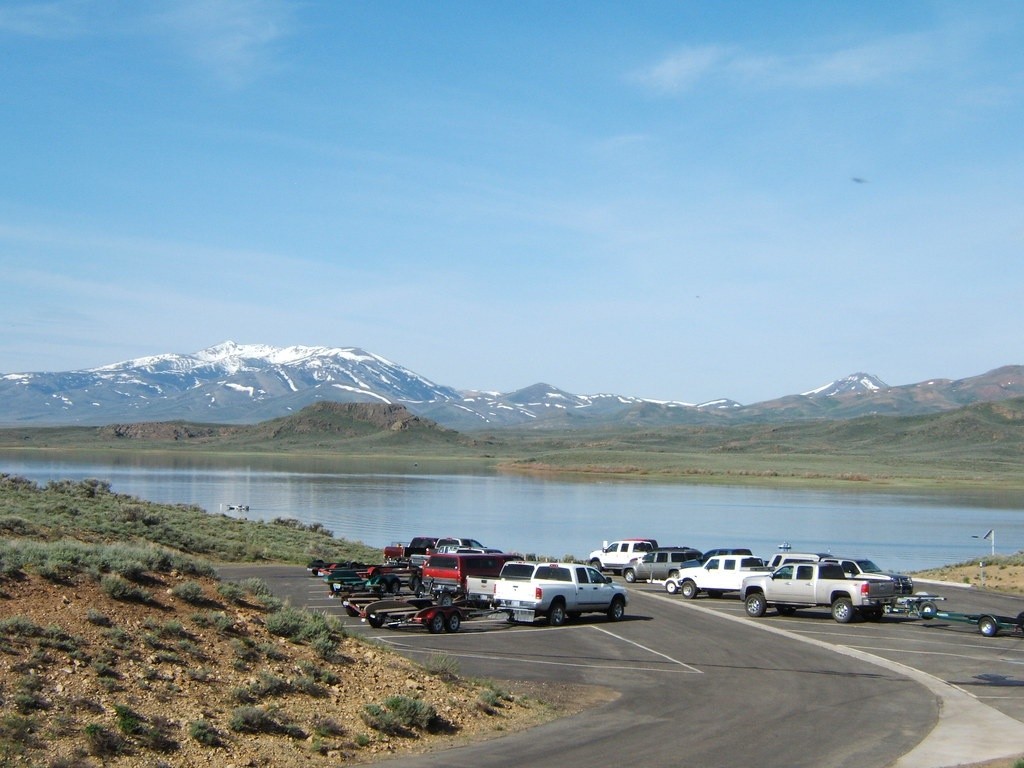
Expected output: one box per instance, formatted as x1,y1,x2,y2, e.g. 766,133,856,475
622,547,703,584
422,553,524,596
589,539,658,574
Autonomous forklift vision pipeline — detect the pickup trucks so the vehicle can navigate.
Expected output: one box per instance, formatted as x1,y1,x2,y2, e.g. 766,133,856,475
680,548,769,569
740,562,898,624
464,560,544,601
678,555,794,599
490,563,629,627
766,553,913,597
384,537,503,566
